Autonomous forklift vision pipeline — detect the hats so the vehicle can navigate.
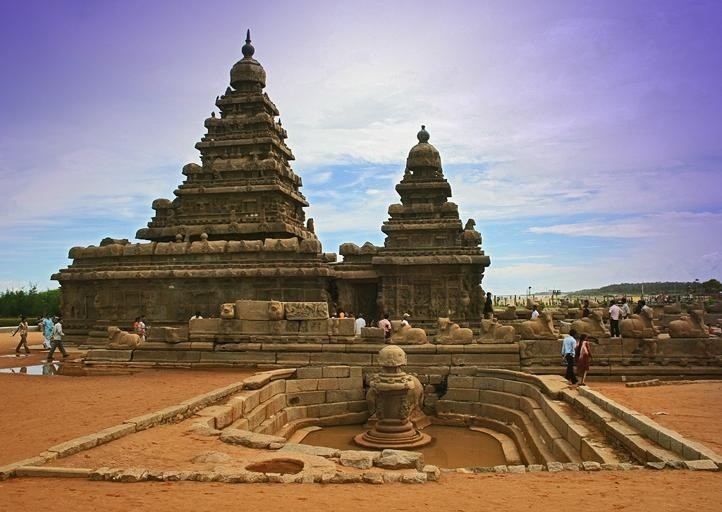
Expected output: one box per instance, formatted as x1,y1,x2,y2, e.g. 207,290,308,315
403,312,411,318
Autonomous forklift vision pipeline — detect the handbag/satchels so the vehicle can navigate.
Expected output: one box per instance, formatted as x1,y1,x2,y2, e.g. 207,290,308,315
580,340,590,361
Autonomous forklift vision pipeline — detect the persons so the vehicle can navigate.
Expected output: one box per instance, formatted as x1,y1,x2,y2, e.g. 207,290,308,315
530,305,540,321
338,309,411,338
483,292,493,318
561,295,646,385
134,315,149,342
190,311,203,320
11,315,70,363
